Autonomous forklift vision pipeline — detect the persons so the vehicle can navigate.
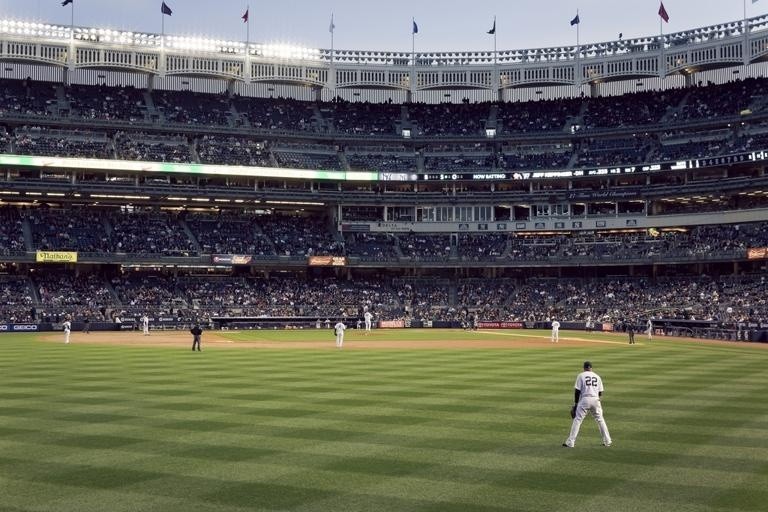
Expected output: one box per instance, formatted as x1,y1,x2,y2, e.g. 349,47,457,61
562,361,614,448
550,318,561,344
0,75,766,192
2,200,766,341
334,318,346,347
189,324,205,351
627,325,636,344
62,317,72,345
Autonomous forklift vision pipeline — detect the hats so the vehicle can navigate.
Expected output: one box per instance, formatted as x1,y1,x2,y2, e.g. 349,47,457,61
584,361,592,368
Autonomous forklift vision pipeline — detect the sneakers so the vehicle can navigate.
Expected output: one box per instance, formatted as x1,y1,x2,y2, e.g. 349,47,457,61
563,444,568,447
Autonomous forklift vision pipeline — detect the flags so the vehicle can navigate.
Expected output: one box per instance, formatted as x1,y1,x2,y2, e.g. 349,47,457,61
62,0,72,7
413,21,418,34
161,2,173,17
571,16,579,26
487,21,495,34
329,17,335,34
241,10,248,23
658,3,669,23
